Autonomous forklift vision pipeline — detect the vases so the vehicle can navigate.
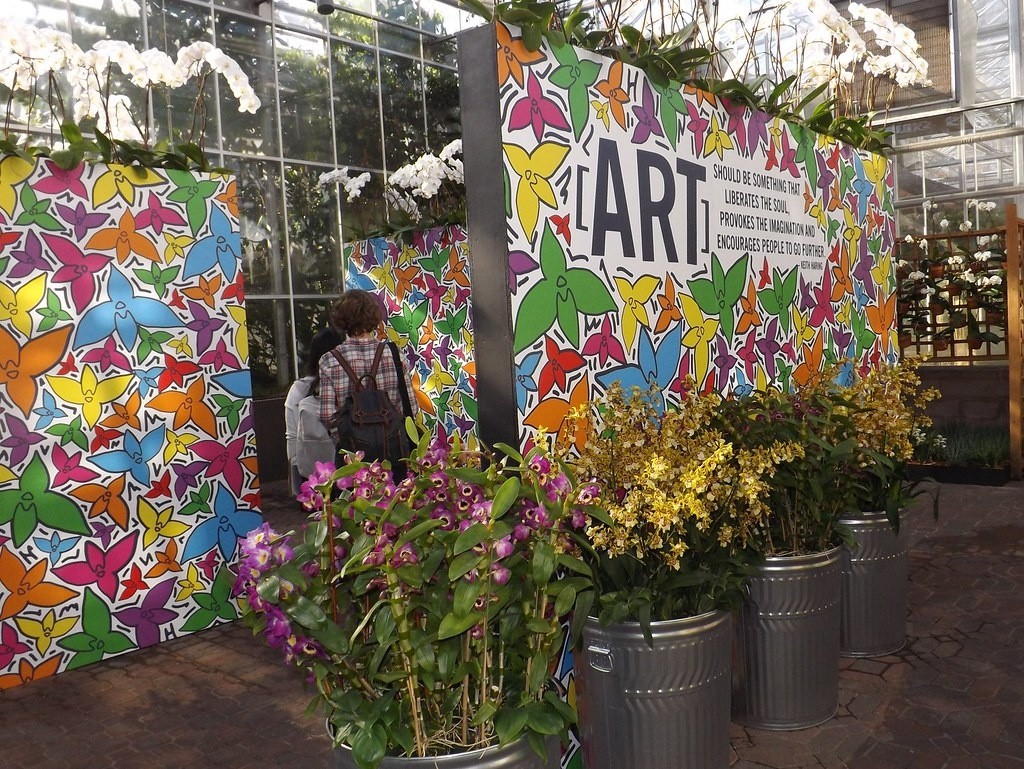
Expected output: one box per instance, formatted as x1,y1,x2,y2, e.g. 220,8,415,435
929,263,945,278
836,507,909,661
964,264,978,276
324,676,562,769
581,610,734,769
897,303,910,316
929,304,945,315
721,539,843,732
966,297,984,309
1000,262,1007,270
986,312,1003,324
945,284,962,296
896,268,909,279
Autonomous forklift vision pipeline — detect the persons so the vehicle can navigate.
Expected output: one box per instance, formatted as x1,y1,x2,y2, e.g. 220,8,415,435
284,328,344,514
318,289,419,502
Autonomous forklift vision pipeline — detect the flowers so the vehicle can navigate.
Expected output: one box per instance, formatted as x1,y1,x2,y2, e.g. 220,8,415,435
0,0,1008,769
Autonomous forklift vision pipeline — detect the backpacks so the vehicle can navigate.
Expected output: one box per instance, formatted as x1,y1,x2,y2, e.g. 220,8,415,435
327,342,410,471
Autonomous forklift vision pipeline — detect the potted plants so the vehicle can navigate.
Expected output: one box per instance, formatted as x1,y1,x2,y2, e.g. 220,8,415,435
898,326,912,349
913,326,955,351
966,312,1002,349
903,311,930,331
947,306,967,328
